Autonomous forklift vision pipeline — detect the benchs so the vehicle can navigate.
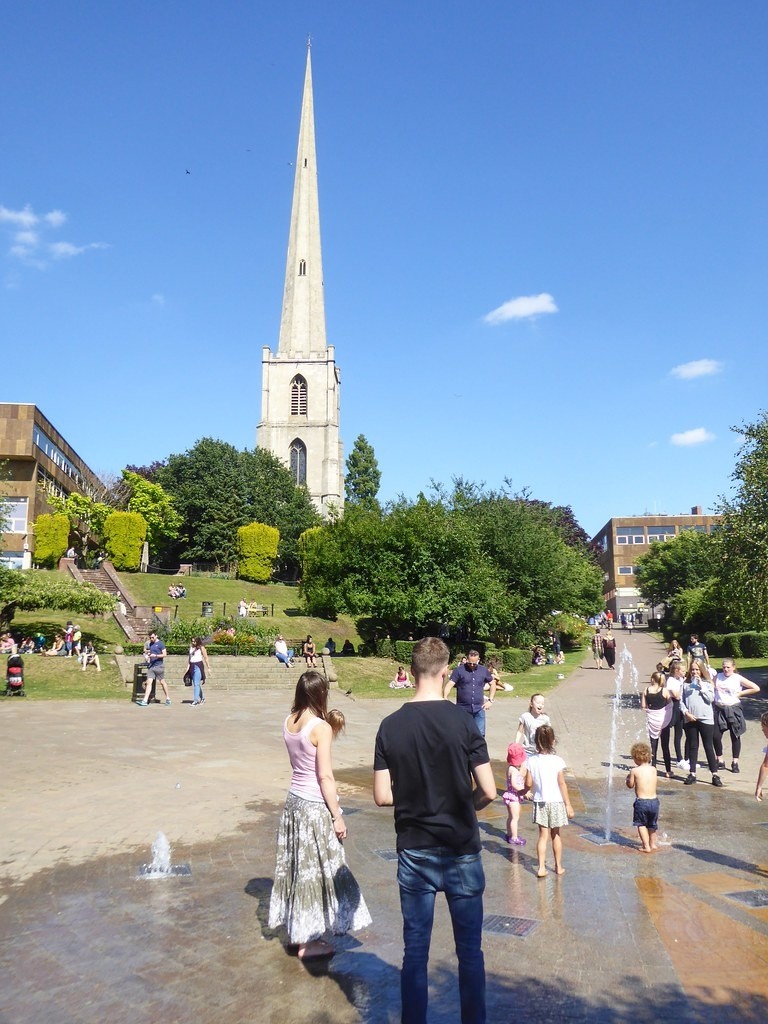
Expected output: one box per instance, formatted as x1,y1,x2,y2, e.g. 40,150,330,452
237,604,268,617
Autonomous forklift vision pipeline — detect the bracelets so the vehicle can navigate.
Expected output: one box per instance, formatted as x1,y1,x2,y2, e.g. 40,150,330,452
489,699,494,703
332,811,340,821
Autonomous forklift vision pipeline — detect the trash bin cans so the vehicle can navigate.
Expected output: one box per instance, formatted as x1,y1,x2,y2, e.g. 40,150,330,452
132,662,156,703
202,601,214,617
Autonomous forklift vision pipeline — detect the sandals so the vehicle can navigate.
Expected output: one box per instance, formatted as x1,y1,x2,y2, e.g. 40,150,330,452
505,833,526,845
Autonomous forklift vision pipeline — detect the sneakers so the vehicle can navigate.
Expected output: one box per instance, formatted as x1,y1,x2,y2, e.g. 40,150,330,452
731,761,739,773
712,775,722,787
718,760,725,770
684,775,696,785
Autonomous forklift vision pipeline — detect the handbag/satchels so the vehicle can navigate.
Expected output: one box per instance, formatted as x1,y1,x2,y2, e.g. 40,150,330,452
184,674,192,686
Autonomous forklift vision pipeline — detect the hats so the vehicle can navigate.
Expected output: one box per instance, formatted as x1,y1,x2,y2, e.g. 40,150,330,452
66,621,72,625
74,625,80,630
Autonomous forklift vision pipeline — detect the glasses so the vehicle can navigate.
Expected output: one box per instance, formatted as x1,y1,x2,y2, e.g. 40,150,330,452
467,659,479,666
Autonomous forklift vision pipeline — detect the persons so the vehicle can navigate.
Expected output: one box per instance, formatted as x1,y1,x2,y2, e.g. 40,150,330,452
176,582,186,599
275,635,355,668
188,636,212,707
19,637,35,653
591,609,634,669
74,625,81,656
488,663,500,683
61,621,74,657
458,657,467,666
0,634,15,653
144,639,150,663
168,582,179,599
374,637,575,1024
67,547,105,570
239,597,257,617
626,633,760,853
394,666,409,688
36,633,46,651
136,630,171,705
755,712,768,801
80,640,101,672
42,634,67,656
268,669,373,959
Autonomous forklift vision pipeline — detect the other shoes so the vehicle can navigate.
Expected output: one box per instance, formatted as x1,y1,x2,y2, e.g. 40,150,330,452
191,701,198,706
686,760,700,769
676,759,691,771
136,700,148,706
200,699,204,705
666,772,676,778
166,700,171,706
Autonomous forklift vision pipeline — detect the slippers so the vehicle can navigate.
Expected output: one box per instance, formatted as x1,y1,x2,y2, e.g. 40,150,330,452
298,941,336,960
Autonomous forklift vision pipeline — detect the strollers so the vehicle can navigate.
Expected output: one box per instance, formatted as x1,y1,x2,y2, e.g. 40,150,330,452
3,655,27,696
